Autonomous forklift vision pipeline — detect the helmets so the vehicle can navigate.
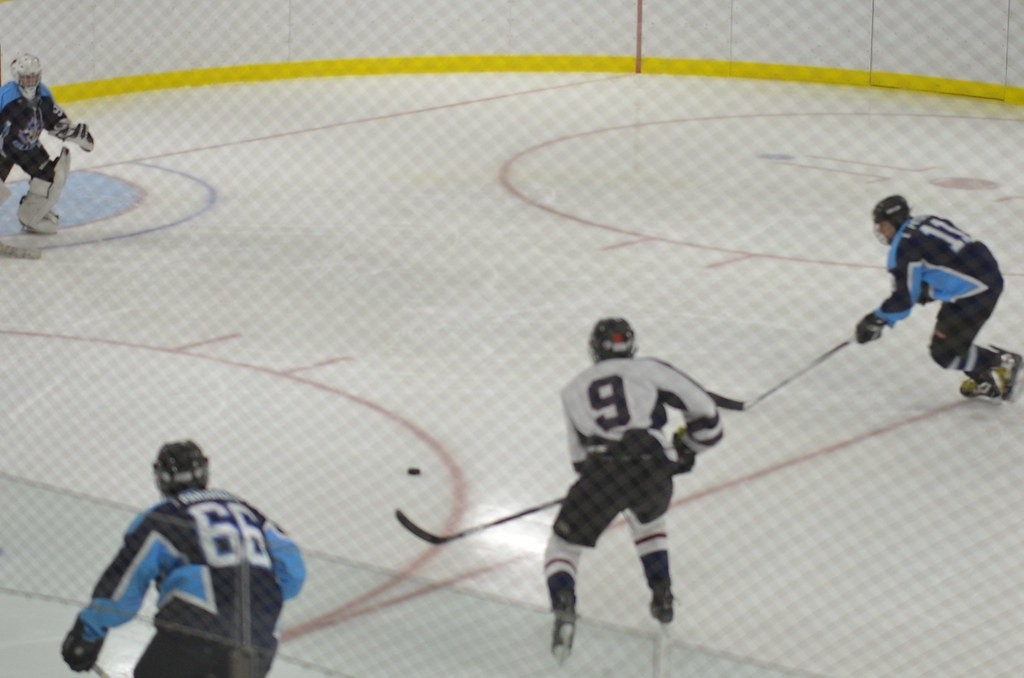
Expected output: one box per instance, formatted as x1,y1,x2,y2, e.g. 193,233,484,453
589,317,635,359
872,195,910,245
10,53,43,101
154,440,208,493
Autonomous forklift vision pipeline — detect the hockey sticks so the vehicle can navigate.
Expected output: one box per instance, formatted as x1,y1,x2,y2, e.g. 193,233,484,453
393,456,684,548
705,317,890,412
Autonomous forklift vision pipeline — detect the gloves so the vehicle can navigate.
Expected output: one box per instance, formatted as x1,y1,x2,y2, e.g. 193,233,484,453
60,620,103,673
671,433,696,472
856,313,888,343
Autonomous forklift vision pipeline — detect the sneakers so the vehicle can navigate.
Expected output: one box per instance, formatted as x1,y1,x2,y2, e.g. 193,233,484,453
960,378,998,399
991,345,1024,402
650,580,676,632
551,591,577,666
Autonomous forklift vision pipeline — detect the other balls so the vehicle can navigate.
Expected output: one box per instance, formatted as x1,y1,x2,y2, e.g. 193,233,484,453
408,468,420,474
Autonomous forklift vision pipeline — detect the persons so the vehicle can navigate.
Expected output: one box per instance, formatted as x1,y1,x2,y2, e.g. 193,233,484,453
0,54,94,234
544,318,723,665
61,440,305,678
857,196,1024,406
